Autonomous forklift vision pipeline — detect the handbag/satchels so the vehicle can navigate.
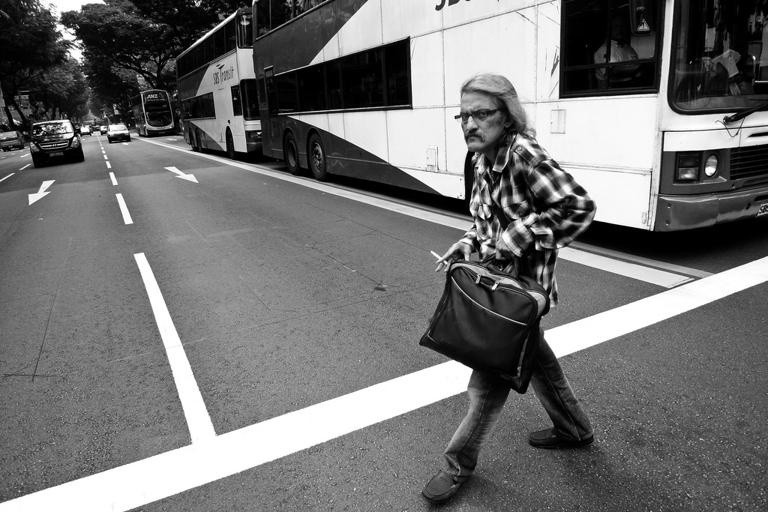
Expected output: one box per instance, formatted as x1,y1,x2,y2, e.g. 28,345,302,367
419,261,550,394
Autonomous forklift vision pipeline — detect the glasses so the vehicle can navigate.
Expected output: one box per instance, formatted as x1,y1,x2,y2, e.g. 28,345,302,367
455,107,502,121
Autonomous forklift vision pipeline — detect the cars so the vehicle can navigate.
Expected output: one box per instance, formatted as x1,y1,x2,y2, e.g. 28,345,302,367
74,120,131,144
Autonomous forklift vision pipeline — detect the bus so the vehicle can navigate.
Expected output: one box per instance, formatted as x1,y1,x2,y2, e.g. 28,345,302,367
248,2,767,246
173,7,262,161
129,88,175,137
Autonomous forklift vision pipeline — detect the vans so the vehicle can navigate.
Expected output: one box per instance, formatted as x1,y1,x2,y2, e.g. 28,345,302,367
28,119,84,168
0,130,25,152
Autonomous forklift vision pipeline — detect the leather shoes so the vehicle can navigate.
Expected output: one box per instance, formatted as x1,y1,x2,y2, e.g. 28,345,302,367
529,428,594,449
421,470,469,502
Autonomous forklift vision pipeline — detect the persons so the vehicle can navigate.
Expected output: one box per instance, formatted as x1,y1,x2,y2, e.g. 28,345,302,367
417,72,594,504
593,15,652,89
